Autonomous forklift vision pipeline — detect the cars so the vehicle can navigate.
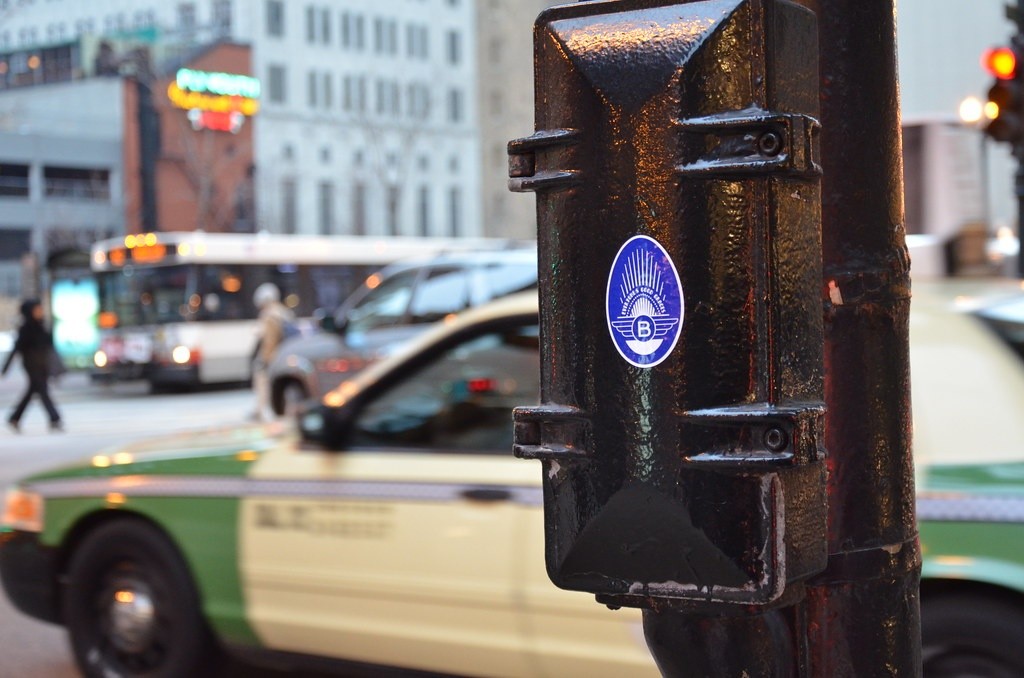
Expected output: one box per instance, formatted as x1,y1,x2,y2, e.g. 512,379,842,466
0,283,1024,678
266,251,541,414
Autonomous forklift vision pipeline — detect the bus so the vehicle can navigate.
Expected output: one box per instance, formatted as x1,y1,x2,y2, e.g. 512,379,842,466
86,229,506,390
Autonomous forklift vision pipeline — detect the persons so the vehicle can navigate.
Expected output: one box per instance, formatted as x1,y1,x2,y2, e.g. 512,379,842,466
1,296,62,432
251,283,295,421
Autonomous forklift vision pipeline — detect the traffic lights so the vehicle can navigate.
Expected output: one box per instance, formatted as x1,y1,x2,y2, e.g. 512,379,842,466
981,40,1019,143
